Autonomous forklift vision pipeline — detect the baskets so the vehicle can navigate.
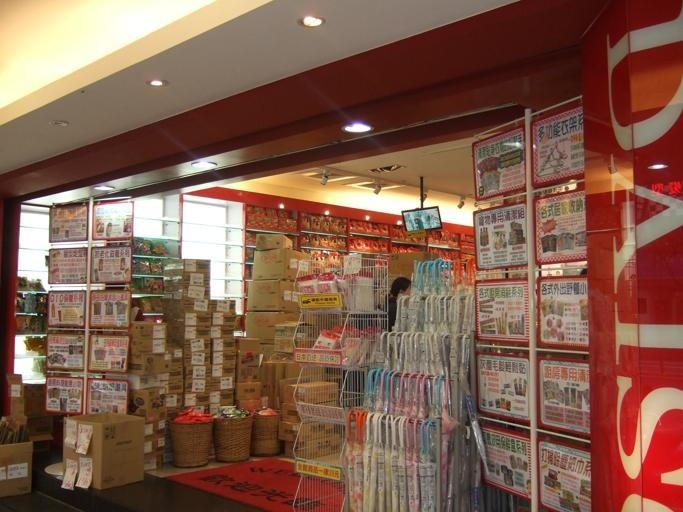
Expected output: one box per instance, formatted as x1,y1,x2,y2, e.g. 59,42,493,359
166,413,214,468
250,411,282,457
213,408,253,461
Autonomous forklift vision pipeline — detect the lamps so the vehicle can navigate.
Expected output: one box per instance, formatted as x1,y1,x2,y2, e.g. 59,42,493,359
320,173,464,209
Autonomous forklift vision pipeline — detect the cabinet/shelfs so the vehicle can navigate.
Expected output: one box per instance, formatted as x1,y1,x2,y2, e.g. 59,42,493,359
12,291,48,360
291,257,390,512
131,234,181,322
225,200,474,315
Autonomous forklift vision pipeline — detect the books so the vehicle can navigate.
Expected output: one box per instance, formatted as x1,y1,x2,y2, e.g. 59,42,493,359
0,417,29,445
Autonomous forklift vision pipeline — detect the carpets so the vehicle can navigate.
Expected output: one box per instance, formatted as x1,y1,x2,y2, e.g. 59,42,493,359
163,457,349,510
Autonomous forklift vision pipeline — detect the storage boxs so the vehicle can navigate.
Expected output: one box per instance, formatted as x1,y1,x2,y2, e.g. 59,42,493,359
131,259,261,471
0,373,55,498
62,412,145,490
244,232,440,459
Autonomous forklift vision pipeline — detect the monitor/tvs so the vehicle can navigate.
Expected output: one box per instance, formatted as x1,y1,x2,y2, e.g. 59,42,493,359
401,205,442,232
